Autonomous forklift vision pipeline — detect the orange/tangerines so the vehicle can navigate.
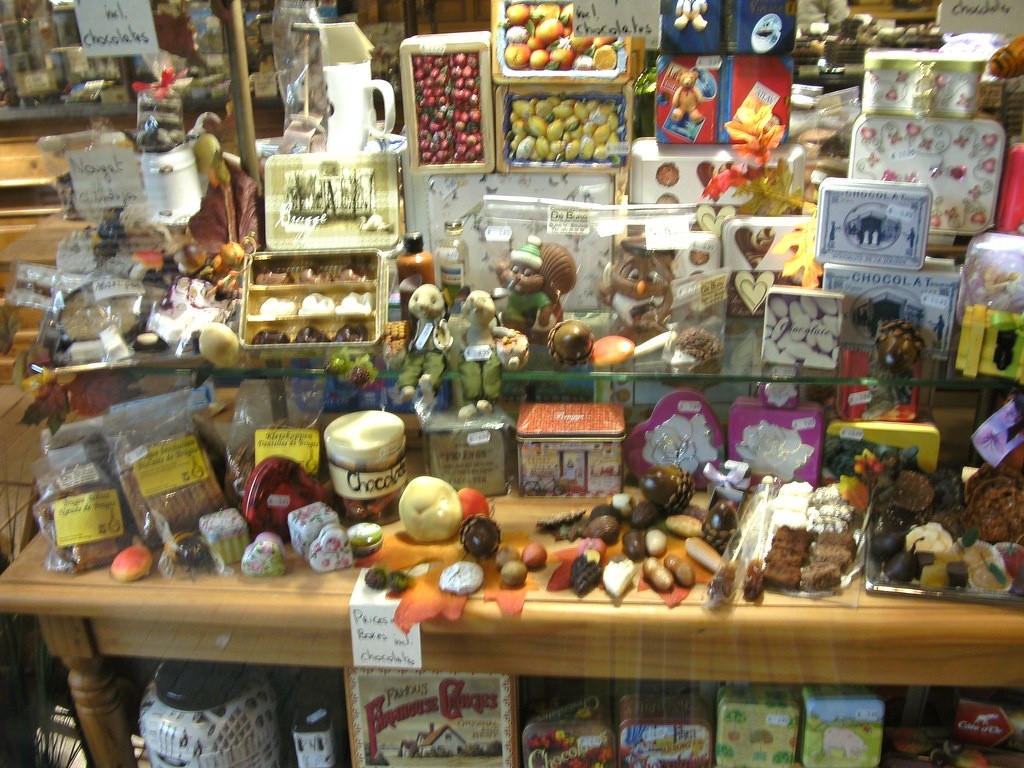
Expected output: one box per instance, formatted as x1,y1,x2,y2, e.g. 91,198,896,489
504,4,620,71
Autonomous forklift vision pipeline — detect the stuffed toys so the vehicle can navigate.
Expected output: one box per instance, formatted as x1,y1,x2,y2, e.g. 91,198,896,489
458,290,515,418
397,283,459,402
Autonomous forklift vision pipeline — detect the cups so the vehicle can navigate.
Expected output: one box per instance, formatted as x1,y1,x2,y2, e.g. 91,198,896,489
142,147,200,214
324,60,396,152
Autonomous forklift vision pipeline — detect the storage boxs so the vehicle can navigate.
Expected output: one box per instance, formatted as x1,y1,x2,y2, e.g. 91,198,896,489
311,521,354,572
324,411,409,527
286,502,343,562
949,686,1024,754
244,454,328,543
291,707,338,768
953,231,1024,327
822,257,961,362
832,342,920,424
861,51,985,120
622,388,726,491
616,690,712,766
846,113,1005,235
821,419,941,489
239,530,286,576
343,670,520,768
814,176,932,270
230,1,812,367
423,410,507,498
344,521,382,557
713,680,801,768
761,284,845,370
727,395,822,489
797,683,884,767
522,695,614,768
199,506,253,566
513,401,626,500
954,305,1023,388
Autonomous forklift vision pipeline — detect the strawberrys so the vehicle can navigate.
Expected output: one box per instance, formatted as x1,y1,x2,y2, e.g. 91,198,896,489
411,53,484,165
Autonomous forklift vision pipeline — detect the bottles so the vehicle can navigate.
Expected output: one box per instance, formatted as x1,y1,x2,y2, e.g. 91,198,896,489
438,221,469,293
397,230,435,321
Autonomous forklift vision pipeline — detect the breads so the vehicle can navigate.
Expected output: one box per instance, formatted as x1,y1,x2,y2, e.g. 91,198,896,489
32,430,226,573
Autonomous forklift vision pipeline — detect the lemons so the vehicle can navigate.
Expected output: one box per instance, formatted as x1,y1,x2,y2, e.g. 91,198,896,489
508,98,624,162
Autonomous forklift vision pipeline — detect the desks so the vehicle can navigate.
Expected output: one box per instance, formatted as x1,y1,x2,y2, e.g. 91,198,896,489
0,483,1024,767
79,289,1024,482
